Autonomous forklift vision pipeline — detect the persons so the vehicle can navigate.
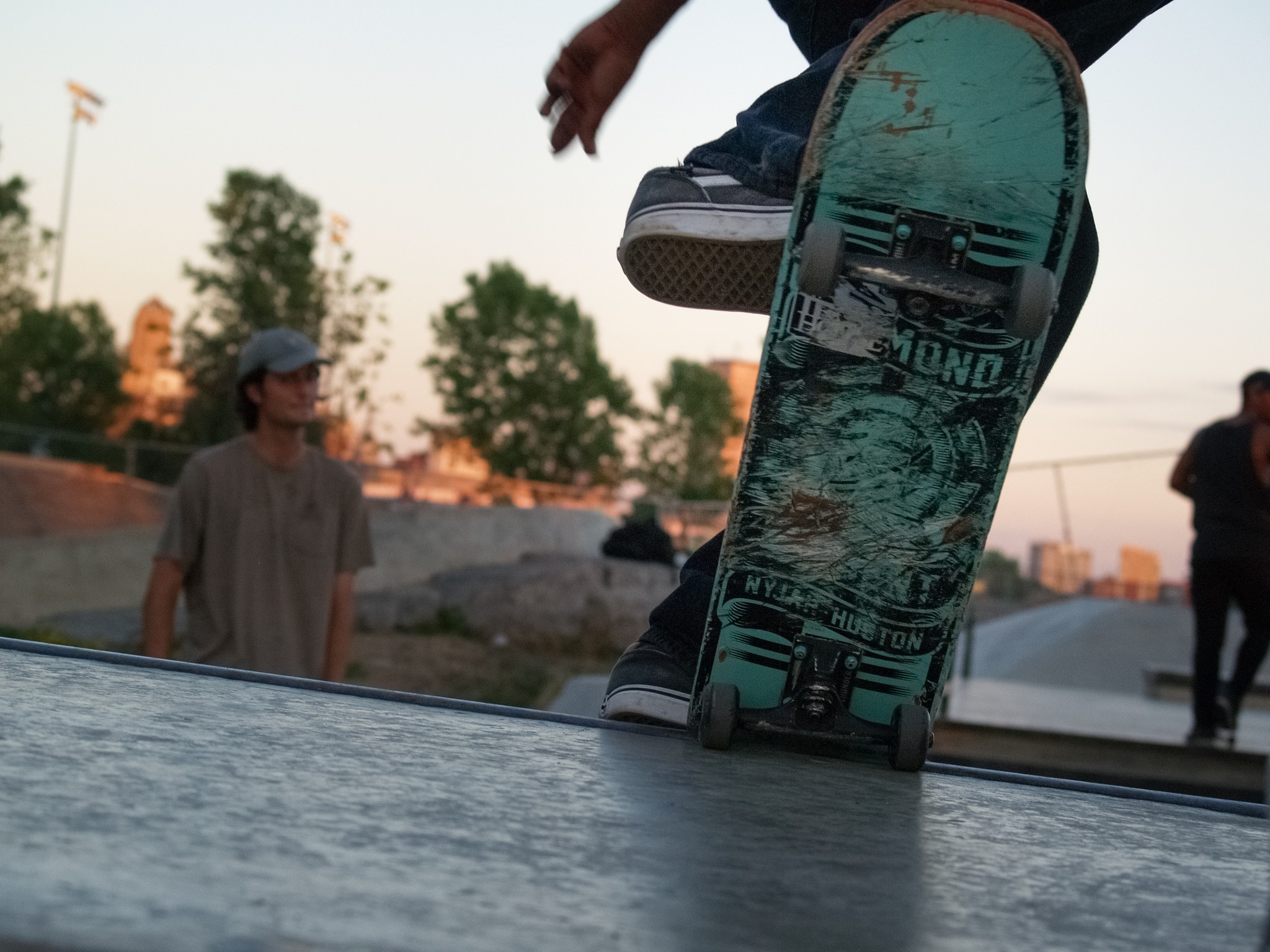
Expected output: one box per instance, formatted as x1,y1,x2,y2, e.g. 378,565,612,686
1167,371,1270,745
536,0,1175,730
142,328,373,687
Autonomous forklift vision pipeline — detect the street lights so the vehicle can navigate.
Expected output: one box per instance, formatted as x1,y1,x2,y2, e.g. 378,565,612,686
43,77,107,329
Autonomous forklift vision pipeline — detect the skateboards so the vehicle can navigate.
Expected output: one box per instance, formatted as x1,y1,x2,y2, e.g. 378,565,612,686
1195,699,1238,747
685,1,1090,774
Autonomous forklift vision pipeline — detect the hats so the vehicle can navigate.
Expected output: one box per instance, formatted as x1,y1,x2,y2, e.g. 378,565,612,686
235,327,336,386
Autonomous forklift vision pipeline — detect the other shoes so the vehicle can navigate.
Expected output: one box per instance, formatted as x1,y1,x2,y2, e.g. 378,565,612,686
1184,722,1235,750
616,166,800,314
598,637,693,723
